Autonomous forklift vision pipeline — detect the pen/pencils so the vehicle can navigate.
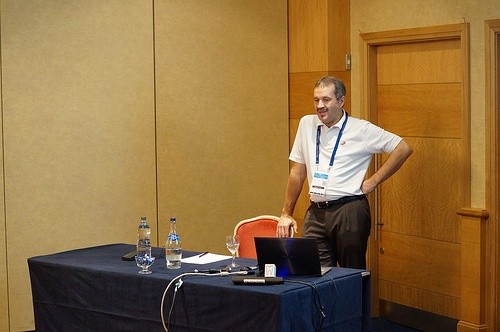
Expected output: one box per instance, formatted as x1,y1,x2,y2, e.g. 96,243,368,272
229,271,255,274
199,251,209,257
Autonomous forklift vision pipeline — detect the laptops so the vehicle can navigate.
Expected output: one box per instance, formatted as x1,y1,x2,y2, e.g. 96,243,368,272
253,237,332,277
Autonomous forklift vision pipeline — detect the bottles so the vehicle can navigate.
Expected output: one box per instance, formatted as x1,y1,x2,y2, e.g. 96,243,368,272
165,217,181,260
137,216,151,257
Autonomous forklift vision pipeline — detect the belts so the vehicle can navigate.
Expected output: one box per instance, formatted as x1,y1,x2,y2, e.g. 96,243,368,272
310,194,366,209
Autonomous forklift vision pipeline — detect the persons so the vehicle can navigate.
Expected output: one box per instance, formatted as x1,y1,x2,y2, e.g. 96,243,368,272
276,77,413,269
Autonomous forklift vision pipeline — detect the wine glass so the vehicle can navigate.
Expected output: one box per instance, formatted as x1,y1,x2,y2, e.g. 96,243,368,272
225,235,240,268
135,256,155,275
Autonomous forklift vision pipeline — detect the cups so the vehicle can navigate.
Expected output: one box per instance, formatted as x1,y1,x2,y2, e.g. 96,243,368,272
166,237,182,269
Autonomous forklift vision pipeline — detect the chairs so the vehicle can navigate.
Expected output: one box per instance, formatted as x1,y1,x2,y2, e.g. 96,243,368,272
233,214,294,258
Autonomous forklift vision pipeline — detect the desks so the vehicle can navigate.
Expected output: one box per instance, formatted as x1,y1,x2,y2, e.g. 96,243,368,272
28,243,371,332
